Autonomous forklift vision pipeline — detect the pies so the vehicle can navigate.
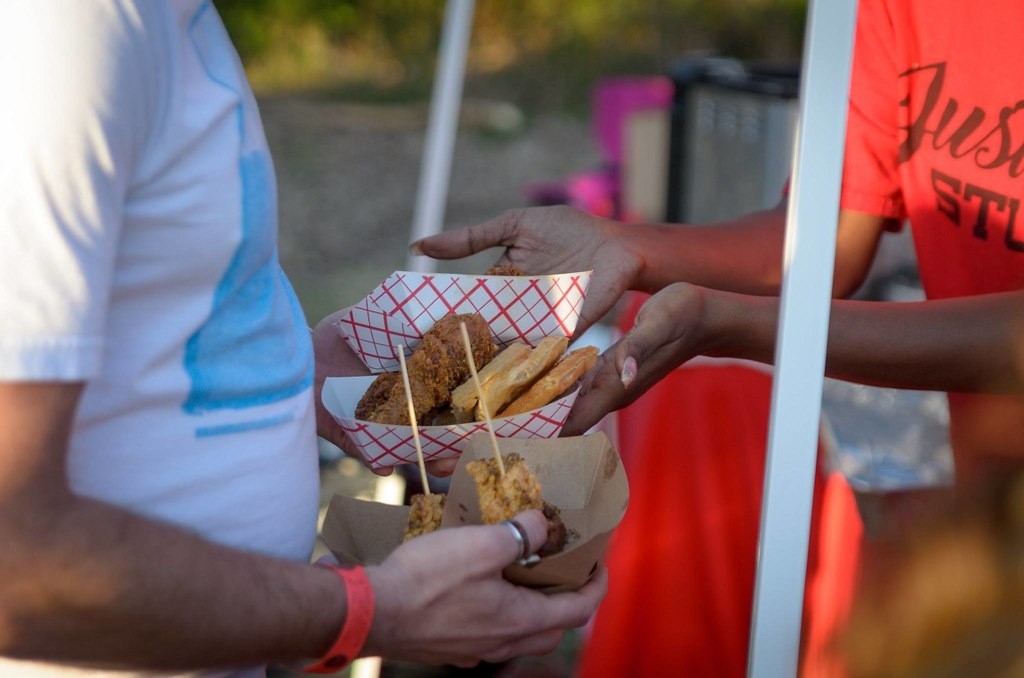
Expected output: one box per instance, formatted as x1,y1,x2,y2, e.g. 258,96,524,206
446,334,601,421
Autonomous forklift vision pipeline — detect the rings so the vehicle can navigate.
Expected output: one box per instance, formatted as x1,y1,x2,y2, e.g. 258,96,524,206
499,520,541,568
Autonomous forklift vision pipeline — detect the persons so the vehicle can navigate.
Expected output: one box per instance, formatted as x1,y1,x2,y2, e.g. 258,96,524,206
0,0,608,678
406,0,1024,678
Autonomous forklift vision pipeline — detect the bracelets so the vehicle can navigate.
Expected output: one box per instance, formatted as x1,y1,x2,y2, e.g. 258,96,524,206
298,562,375,675
